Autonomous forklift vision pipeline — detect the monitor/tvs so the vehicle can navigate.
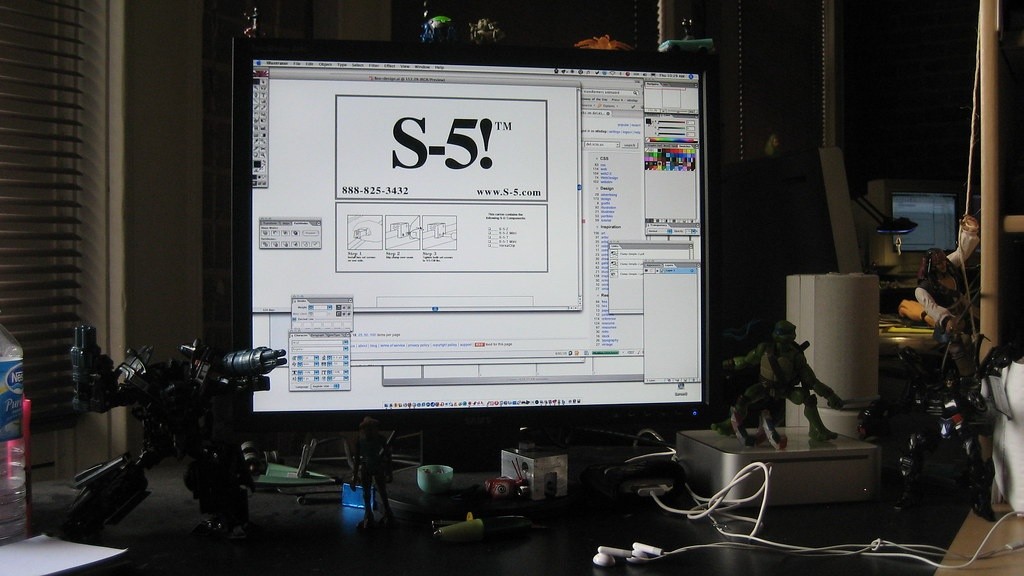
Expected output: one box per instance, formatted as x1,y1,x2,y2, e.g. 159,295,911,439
219,36,732,523
889,190,958,252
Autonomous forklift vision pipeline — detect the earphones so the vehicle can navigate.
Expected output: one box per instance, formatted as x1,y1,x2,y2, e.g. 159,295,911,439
592,545,633,566
626,542,664,565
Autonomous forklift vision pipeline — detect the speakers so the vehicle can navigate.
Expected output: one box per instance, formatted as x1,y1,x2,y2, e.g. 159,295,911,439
780,272,885,438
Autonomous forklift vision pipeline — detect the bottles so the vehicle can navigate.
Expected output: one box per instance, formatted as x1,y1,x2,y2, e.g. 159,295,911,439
0,323,28,543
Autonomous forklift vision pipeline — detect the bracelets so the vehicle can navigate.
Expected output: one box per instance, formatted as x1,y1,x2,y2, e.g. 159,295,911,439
921,311,927,322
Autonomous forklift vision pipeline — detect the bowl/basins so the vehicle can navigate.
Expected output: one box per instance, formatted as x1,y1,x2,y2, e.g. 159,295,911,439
417,465,454,494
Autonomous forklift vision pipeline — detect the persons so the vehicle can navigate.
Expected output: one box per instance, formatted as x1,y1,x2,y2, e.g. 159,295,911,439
711,320,845,450
350,416,394,529
856,323,1011,522
898,299,937,329
46,325,287,542
915,213,989,413
